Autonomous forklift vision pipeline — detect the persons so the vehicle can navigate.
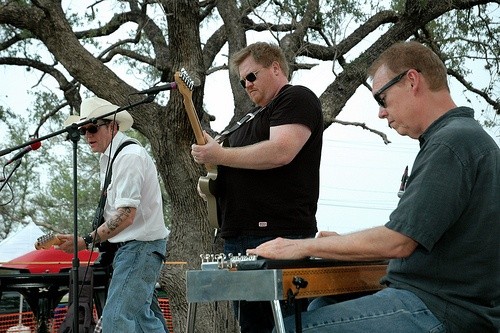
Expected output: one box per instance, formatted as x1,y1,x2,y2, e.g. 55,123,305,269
245,41,500,333
54,98,170,333
190,42,323,333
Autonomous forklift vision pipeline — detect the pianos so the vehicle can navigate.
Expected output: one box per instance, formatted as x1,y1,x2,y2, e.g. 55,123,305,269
185,250,388,333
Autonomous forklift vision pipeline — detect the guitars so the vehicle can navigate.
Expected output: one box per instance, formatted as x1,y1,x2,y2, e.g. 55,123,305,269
174,67,225,230
34,231,117,252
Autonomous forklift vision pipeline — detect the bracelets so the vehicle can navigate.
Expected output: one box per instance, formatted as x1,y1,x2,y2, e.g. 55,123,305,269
82,234,94,250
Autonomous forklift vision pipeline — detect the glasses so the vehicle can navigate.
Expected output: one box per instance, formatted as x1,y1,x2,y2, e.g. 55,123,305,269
78,123,109,135
240,63,268,88
374,68,421,108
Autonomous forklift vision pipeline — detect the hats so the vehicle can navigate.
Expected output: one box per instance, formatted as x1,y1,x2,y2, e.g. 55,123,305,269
64,96,133,131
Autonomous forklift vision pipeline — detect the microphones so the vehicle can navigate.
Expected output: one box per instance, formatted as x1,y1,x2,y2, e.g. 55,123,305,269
131,82,178,95
397,165,408,197
7,138,41,164
